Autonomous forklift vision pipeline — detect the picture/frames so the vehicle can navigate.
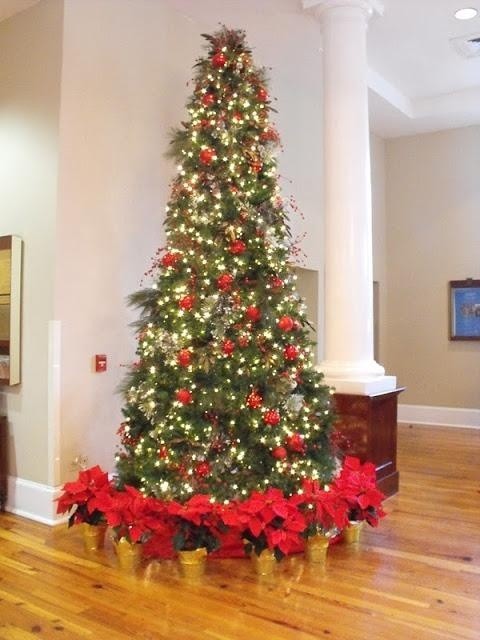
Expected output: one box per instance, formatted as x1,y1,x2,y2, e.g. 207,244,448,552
447,278,480,339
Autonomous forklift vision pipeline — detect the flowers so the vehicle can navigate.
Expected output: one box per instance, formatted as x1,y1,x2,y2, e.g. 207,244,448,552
53,465,213,562
214,478,348,558
79,520,365,579
337,456,387,529
